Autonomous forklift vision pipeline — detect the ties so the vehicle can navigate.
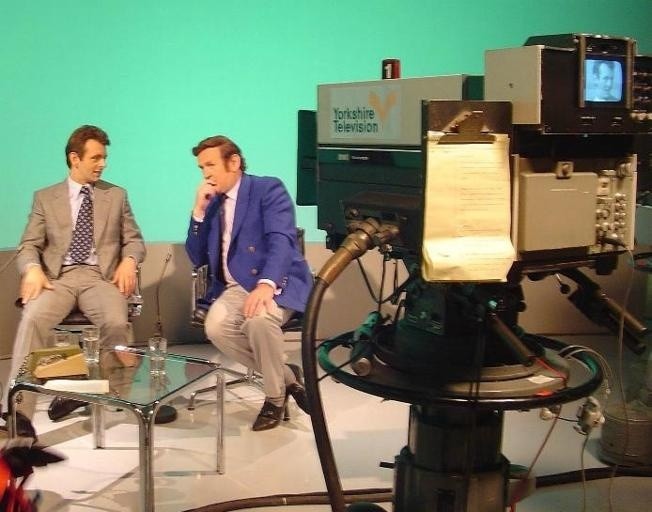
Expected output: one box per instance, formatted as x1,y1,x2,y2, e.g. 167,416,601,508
68,185,99,265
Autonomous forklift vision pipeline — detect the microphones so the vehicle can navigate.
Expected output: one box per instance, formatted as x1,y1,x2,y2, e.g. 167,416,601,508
1,243,25,282
145,253,177,424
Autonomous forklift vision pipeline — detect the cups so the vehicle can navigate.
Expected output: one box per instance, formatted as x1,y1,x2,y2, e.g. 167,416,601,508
83,326,100,364
148,337,167,378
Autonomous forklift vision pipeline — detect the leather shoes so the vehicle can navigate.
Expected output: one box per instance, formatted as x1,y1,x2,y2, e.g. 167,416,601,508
0,414,38,444
48,393,90,421
252,391,290,432
284,363,309,415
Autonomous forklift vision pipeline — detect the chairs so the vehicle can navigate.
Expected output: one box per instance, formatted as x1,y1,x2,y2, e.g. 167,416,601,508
188,229,316,421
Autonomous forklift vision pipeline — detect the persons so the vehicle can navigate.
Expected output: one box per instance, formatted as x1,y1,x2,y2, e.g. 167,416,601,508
0,123,146,434
183,135,316,434
